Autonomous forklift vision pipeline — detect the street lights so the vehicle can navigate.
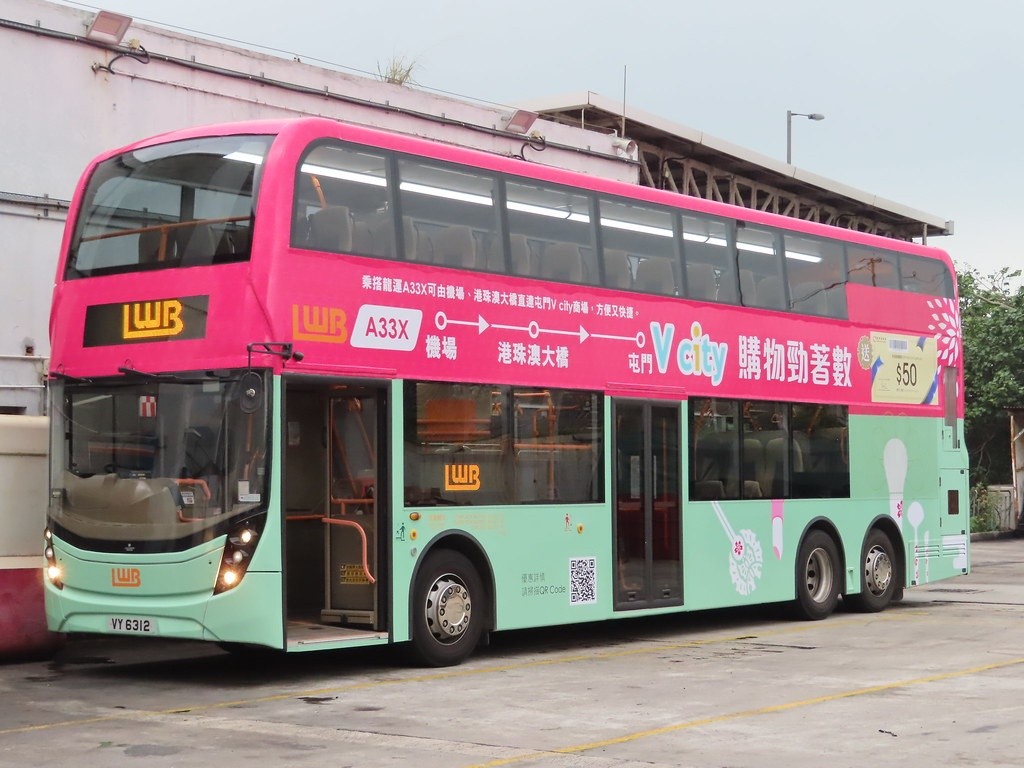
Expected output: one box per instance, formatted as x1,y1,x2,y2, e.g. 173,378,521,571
785,108,825,166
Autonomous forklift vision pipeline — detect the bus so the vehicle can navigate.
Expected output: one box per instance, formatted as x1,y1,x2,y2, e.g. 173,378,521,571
38,113,978,669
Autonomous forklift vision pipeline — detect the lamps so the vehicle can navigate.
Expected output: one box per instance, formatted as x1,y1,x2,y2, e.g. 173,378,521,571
500,107,541,141
82,10,142,49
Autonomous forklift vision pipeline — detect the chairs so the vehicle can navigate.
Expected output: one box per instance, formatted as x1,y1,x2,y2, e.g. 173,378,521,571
422,446,592,507
138,218,251,264
688,428,849,500
294,206,849,322
